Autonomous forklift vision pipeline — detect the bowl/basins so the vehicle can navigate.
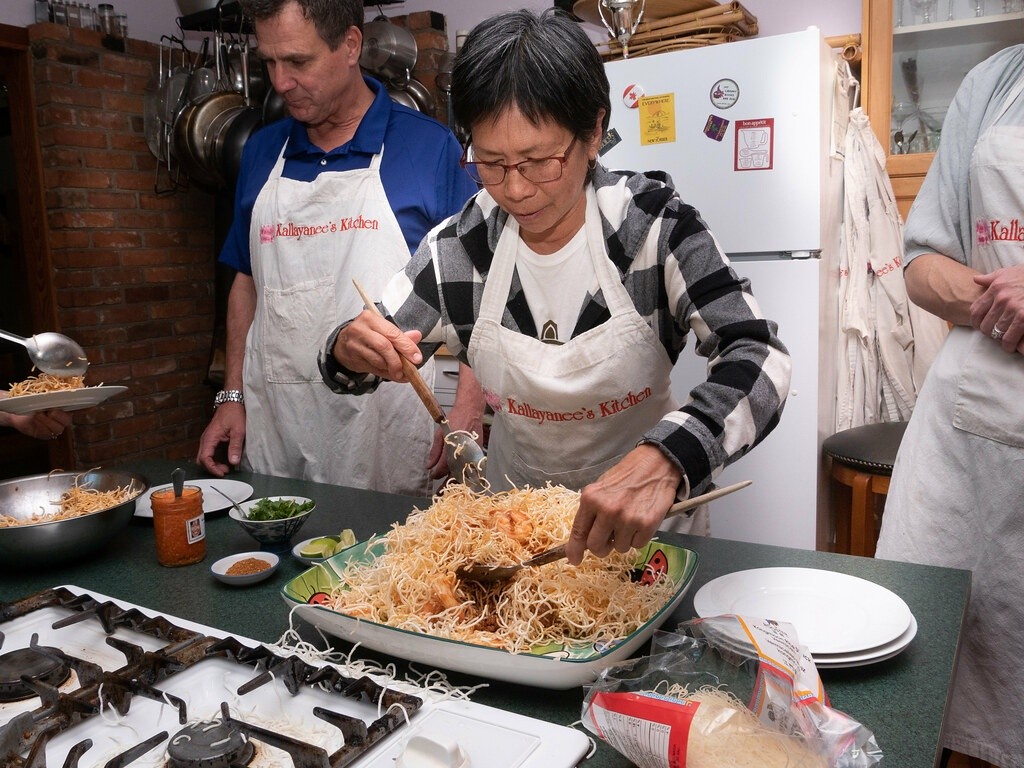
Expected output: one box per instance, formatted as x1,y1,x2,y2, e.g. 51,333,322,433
229,496,316,543
0,468,150,564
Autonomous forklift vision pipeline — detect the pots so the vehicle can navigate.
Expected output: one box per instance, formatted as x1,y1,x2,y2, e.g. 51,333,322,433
173,0,437,184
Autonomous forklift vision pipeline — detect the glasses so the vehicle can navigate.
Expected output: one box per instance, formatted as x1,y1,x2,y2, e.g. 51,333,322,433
460,133,578,186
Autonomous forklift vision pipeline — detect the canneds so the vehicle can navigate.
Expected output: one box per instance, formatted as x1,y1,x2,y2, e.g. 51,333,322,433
150,485,206,568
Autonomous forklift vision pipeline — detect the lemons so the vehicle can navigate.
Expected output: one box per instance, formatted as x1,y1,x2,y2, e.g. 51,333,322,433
300,528,356,558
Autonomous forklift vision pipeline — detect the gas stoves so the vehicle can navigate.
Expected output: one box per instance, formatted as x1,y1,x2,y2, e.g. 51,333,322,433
0,585,590,767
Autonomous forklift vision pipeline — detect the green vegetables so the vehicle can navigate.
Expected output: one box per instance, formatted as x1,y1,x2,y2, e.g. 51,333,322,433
246,497,316,520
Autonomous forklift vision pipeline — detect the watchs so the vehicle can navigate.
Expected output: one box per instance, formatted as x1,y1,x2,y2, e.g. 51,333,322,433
213,388,246,410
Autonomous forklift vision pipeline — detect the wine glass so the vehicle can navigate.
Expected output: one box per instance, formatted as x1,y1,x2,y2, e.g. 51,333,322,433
890,102,948,154
894,0,1024,27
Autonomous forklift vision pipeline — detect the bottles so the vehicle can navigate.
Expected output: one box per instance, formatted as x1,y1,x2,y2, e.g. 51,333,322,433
150,485,207,568
35,0,128,38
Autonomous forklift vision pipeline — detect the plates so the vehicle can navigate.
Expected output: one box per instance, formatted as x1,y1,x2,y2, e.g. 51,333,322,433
133,478,254,518
694,568,919,669
210,552,280,585
292,535,359,564
0,385,129,415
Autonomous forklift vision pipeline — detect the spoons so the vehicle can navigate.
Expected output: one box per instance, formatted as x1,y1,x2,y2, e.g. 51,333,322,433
895,130,918,154
1,329,88,377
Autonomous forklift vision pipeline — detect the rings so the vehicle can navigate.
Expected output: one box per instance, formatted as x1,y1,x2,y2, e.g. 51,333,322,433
991,324,1005,340
49,432,62,440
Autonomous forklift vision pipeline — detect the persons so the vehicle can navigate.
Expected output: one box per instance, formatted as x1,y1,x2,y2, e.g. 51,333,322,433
872,38,1024,768
195,1,487,503
0,389,74,442
315,5,794,567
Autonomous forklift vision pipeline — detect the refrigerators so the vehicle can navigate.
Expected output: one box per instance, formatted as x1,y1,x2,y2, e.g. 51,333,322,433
603,27,855,552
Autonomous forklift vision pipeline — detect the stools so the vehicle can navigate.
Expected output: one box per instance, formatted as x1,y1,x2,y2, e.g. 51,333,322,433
824,422,909,558
